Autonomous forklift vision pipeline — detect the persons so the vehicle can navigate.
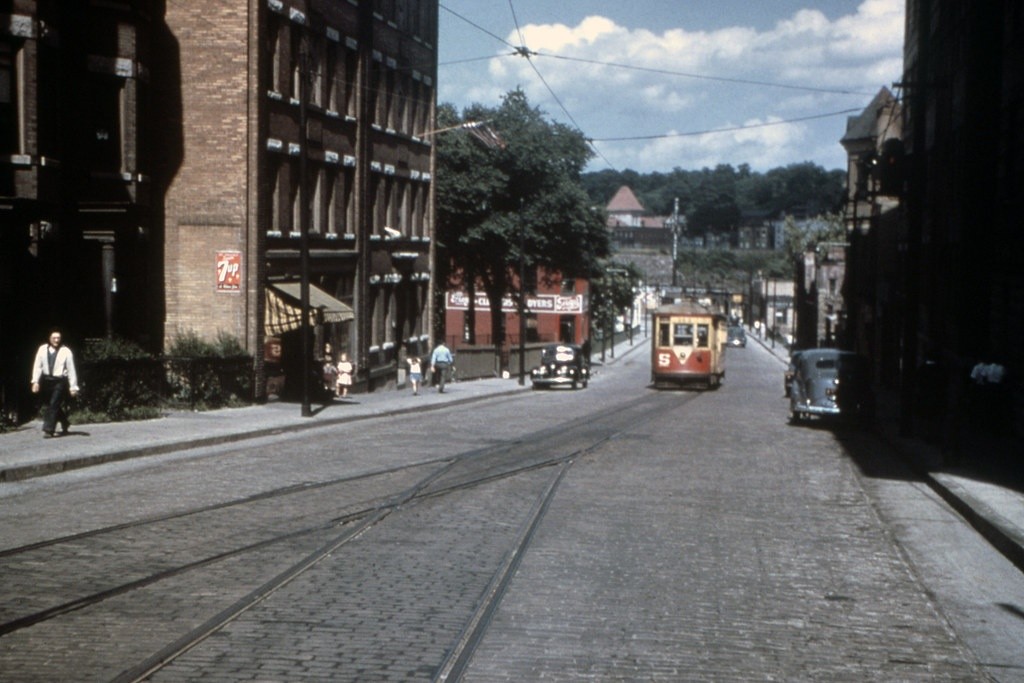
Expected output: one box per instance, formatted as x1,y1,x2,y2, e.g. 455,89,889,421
335,352,353,395
321,355,338,390
430,338,452,393
580,338,591,365
30,328,81,438
406,350,423,395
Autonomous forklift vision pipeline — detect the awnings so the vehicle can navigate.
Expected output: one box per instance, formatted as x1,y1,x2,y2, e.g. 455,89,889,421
264,279,355,339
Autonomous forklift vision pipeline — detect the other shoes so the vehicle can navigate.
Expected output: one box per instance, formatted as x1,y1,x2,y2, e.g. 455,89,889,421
44,433,53,438
62,423,70,433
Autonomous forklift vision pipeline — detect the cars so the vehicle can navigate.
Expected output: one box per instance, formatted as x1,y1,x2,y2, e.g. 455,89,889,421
785,348,863,422
531,344,589,389
727,325,746,348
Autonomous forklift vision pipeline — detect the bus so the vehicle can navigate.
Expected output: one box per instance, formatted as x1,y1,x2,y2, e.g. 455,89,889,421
651,302,728,390
